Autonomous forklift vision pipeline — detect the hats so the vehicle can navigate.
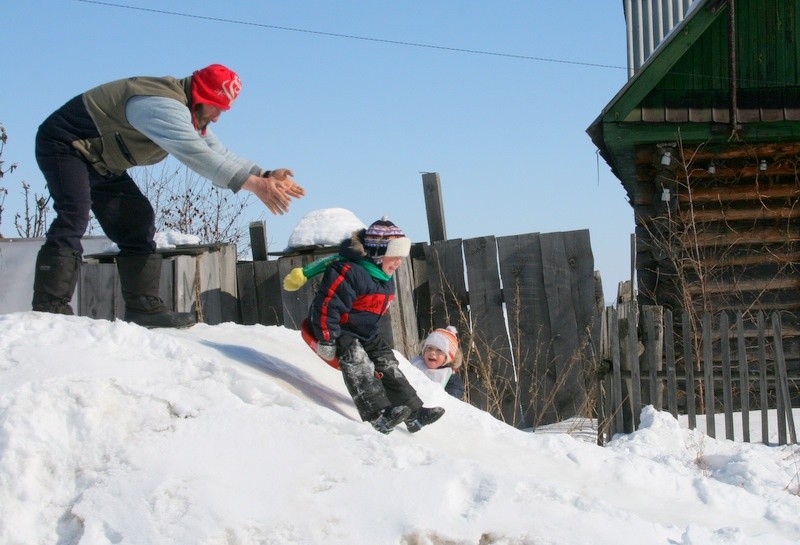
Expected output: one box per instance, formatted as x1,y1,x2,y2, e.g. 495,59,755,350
191,64,241,136
363,216,410,259
426,325,459,367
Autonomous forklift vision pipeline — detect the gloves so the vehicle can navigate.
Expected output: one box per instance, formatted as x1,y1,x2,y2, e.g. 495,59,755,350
316,339,338,362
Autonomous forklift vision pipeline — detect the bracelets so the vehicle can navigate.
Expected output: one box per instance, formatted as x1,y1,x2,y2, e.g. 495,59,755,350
262,170,271,178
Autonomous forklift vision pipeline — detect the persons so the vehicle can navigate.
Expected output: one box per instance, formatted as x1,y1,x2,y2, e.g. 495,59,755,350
31,61,305,330
412,326,466,400
303,218,445,437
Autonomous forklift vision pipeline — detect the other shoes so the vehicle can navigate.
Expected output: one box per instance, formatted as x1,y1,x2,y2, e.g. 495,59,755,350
371,404,410,434
405,407,445,432
124,308,196,328
32,301,76,315
